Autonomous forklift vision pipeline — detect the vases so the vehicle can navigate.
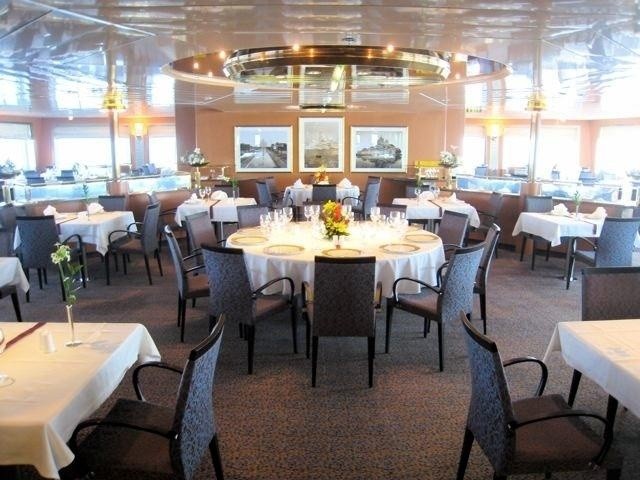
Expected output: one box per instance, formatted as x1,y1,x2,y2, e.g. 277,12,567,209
445,168,452,179
64,305,81,348
193,166,201,189
575,206,579,216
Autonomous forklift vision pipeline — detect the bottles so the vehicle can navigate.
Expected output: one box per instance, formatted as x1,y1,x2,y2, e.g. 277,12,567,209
630,189,637,201
618,188,622,200
39,330,54,355
2,185,15,204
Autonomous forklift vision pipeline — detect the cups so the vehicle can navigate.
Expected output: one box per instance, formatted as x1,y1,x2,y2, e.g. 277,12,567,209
233,185,239,204
24,186,32,201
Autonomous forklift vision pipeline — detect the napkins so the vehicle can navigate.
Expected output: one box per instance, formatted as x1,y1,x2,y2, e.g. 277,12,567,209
42,204,57,217
590,206,608,218
552,203,569,216
86,202,104,216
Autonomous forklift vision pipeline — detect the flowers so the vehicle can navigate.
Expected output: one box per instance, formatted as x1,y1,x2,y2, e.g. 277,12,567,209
571,191,583,205
181,147,209,167
50,241,84,304
441,145,460,167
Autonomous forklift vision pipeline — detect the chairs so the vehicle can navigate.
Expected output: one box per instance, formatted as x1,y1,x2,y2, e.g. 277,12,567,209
384,242,487,370
164,223,240,345
421,223,501,337
198,241,297,371
301,256,382,390
144,173,504,291
16,214,87,302
565,216,639,289
457,311,614,476
520,195,556,273
565,267,639,412
1,255,30,320
67,315,230,478
86,194,127,286
104,201,162,287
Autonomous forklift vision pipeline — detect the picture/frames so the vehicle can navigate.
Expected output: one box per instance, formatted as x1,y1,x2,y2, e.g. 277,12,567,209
233,66,292,104
297,116,345,173
299,65,349,106
350,126,409,174
234,124,293,174
351,64,409,104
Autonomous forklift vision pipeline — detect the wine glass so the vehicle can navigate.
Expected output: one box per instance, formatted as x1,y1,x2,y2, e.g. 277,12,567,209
0,327,14,387
415,189,421,203
260,205,410,249
200,186,211,203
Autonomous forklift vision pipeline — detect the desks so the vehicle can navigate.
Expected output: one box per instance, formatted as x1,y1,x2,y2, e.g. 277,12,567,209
540,318,638,462
13,210,138,290
224,220,445,353
511,208,612,288
